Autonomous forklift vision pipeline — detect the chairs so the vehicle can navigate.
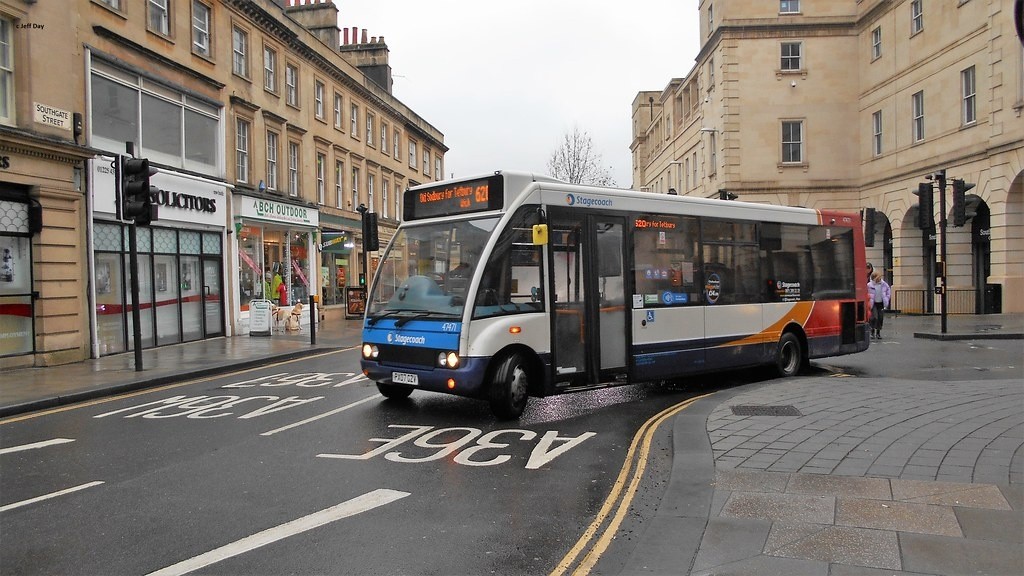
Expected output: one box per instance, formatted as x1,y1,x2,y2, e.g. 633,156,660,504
485,258,501,305
700,257,850,306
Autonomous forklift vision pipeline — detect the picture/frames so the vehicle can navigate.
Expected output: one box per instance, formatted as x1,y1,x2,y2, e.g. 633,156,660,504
0,247,15,282
181,263,192,290
125,263,142,294
155,263,167,292
94,262,111,295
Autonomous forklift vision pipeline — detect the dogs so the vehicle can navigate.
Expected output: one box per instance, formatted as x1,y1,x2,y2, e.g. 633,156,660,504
268,303,290,332
285,303,303,332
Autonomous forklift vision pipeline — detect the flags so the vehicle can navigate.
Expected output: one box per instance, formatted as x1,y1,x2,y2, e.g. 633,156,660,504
319,232,348,252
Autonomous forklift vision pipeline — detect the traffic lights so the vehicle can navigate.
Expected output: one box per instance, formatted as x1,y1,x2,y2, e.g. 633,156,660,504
359,272,366,285
110,153,154,227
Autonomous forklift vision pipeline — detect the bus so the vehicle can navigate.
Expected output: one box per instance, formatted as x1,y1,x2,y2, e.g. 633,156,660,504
361,166,876,421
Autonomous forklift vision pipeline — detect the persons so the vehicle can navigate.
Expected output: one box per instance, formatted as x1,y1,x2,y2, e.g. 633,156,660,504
274,282,288,307
866,271,892,341
864,261,877,288
269,274,284,305
436,242,493,307
